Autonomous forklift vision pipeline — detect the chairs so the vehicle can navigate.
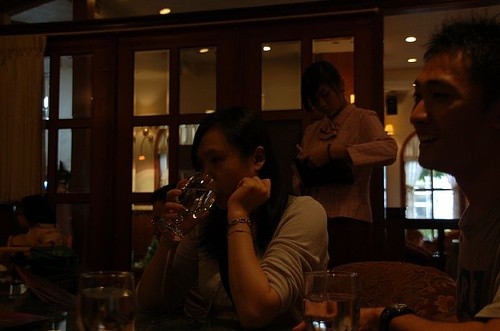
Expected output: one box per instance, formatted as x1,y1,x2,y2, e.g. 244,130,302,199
332,263,456,323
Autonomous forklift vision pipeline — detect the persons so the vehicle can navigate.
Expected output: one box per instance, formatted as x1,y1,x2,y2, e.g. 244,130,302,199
134,107,330,331
293,12,500,331
7,195,73,248
152,184,177,228
294,59,397,269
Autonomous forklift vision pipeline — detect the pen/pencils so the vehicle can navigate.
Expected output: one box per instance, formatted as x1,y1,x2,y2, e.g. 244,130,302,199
297,144,303,152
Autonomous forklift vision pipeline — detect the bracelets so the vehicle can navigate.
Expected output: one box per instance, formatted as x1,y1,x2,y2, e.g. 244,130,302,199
227,229,251,236
227,216,250,228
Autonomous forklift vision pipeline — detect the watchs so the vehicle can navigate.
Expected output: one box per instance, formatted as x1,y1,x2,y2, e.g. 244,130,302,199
378,304,415,331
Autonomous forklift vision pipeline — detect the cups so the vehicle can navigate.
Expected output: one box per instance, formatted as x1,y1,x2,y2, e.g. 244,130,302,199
76,270,136,331
302,270,361,331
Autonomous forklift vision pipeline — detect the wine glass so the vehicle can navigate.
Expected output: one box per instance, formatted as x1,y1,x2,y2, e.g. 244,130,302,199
153,171,218,243
